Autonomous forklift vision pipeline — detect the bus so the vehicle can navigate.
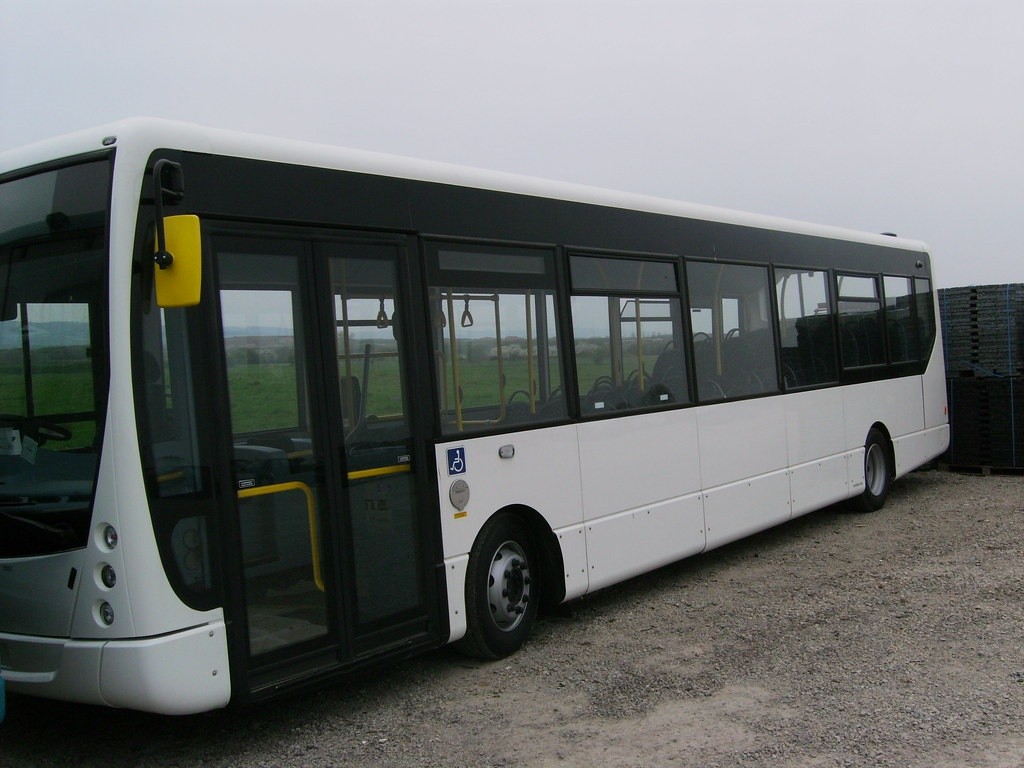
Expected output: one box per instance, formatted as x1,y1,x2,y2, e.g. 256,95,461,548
0,119,951,718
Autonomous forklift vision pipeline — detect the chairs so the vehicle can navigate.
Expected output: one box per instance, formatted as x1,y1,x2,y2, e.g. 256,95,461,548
651,339,684,381
901,315,926,360
506,390,532,423
846,320,873,365
811,321,859,368
693,331,715,380
722,328,756,375
341,375,368,441
887,318,908,362
